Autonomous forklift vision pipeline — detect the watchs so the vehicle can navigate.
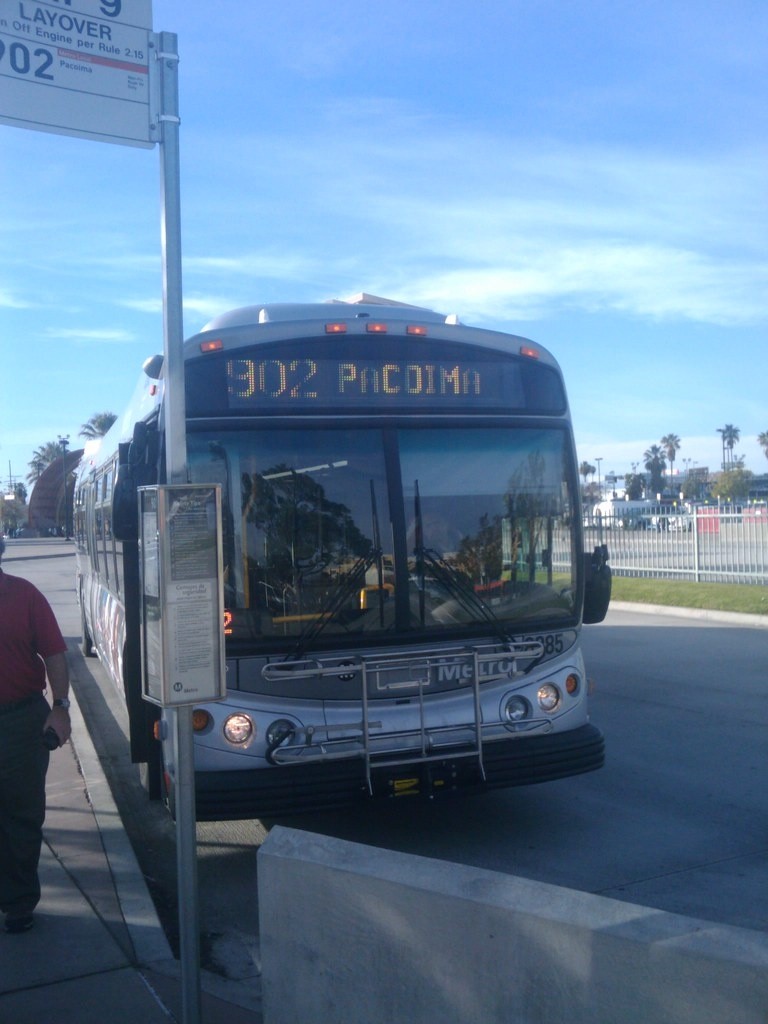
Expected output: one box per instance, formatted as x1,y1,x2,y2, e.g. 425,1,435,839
52,698,71,709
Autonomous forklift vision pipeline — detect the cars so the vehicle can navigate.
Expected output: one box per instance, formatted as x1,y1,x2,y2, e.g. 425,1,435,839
668,517,691,532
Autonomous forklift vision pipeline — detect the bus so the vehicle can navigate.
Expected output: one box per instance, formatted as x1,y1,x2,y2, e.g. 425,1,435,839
70,292,615,826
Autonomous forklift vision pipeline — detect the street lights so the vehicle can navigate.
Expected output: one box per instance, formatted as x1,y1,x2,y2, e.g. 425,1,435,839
716,428,726,472
57,440,73,543
725,446,732,462
595,458,606,503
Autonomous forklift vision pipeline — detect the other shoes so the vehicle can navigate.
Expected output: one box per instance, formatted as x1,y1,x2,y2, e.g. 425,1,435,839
3,912,36,934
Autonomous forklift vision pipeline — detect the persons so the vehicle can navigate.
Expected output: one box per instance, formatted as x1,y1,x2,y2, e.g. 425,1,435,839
1,530,73,933
406,515,467,557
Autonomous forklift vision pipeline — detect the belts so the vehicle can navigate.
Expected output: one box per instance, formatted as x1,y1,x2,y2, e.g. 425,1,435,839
0,698,44,716
